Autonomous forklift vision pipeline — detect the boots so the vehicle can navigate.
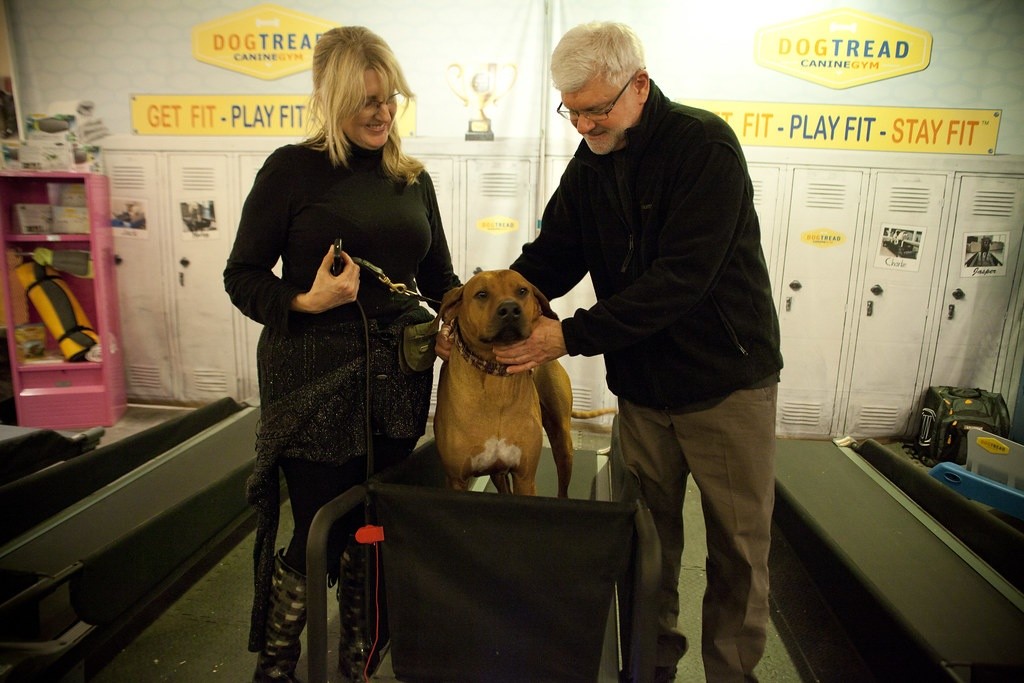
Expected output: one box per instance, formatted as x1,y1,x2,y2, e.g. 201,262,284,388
253,548,307,683
338,530,380,683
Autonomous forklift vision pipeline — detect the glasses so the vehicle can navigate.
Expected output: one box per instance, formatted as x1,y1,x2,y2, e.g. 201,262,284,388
557,67,646,121
360,93,401,114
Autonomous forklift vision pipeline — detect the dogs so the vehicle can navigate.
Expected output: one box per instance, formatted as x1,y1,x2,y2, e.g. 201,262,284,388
427,268,573,498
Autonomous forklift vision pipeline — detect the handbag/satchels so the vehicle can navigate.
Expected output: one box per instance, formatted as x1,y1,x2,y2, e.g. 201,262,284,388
916,385,1011,467
397,318,440,376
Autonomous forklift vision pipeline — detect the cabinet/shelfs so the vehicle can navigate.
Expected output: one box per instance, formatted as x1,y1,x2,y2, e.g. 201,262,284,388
906,169,1023,440
99,150,177,407
830,166,954,439
239,151,285,400
405,153,460,418
744,163,788,302
776,164,874,445
163,151,243,403
0,169,126,430
542,151,609,429
460,153,534,287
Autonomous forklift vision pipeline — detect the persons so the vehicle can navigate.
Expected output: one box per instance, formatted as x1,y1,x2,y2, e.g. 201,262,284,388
223,27,465,683
435,20,784,683
